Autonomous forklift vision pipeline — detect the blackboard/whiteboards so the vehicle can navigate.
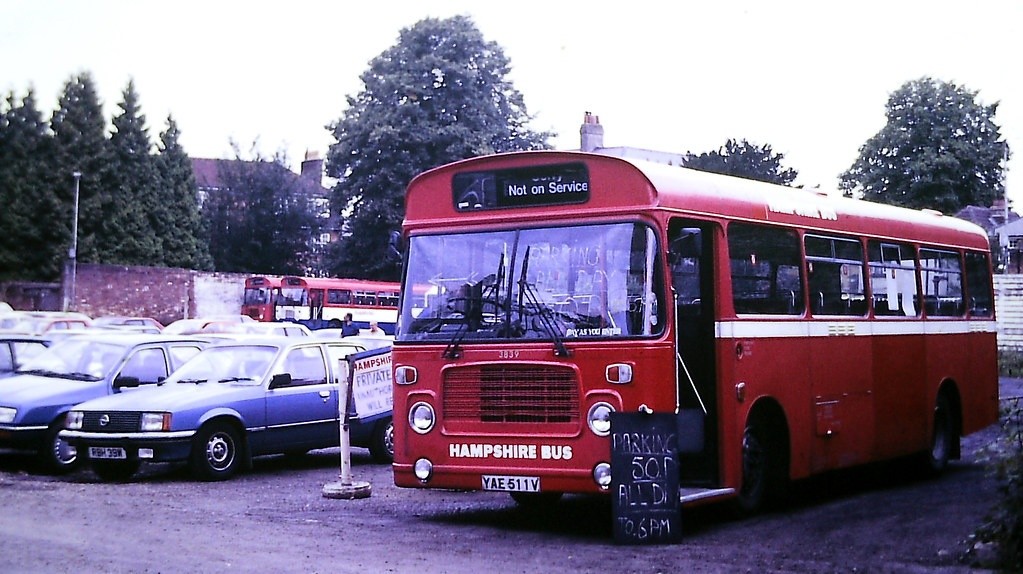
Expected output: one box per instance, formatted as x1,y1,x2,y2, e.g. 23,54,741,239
609,411,680,545
346,344,393,424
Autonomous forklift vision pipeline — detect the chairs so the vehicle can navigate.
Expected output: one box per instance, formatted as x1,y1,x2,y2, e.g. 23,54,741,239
878,293,976,317
244,359,267,378
140,354,164,381
769,289,794,314
94,352,124,379
344,299,394,306
850,293,877,315
794,292,823,315
821,293,850,314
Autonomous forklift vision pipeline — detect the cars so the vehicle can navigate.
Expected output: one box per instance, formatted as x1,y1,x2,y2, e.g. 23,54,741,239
0,302,372,379
0,334,210,473
59,338,394,482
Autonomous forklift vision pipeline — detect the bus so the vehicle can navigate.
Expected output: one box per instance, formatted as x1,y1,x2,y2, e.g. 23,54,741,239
275,276,401,329
383,149,1000,512
241,276,282,322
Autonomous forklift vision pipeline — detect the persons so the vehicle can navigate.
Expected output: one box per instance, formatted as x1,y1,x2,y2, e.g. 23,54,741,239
366,320,385,336
340,313,360,338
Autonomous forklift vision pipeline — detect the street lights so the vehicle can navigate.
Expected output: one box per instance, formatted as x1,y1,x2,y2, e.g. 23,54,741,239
72,171,81,311
